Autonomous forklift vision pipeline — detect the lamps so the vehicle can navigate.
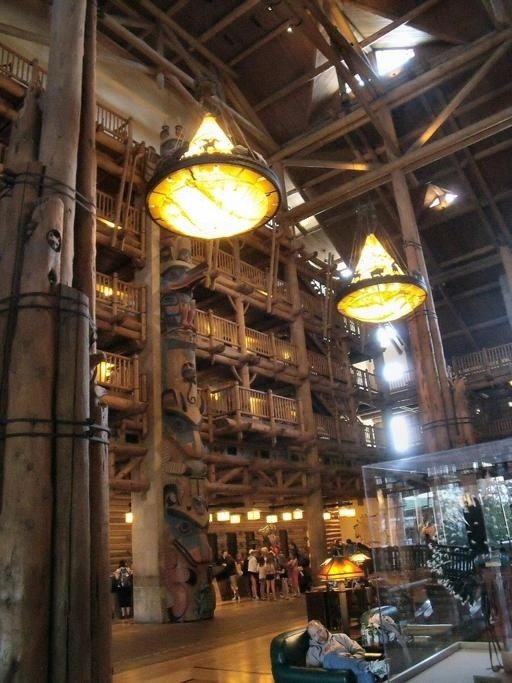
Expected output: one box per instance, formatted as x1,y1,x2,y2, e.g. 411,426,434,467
318,550,365,640
209,505,357,523
143,62,284,239
125,503,132,524
334,191,428,326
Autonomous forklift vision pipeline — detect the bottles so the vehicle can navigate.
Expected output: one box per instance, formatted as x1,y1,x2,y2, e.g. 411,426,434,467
361,623,380,646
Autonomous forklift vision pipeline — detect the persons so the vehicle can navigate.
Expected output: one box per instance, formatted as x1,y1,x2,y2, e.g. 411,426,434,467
344,537,353,554
113,558,135,620
357,542,370,554
305,615,397,683
247,541,311,600
220,550,245,603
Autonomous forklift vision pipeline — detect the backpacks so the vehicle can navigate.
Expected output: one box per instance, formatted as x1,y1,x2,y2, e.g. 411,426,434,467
118,568,131,589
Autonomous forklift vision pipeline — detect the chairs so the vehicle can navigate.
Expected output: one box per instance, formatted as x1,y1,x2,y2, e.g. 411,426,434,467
270,605,452,683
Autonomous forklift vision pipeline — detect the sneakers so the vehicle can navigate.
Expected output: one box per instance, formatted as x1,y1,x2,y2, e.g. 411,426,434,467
232,595,240,600
280,593,300,599
252,596,276,601
120,615,131,619
369,658,391,678
